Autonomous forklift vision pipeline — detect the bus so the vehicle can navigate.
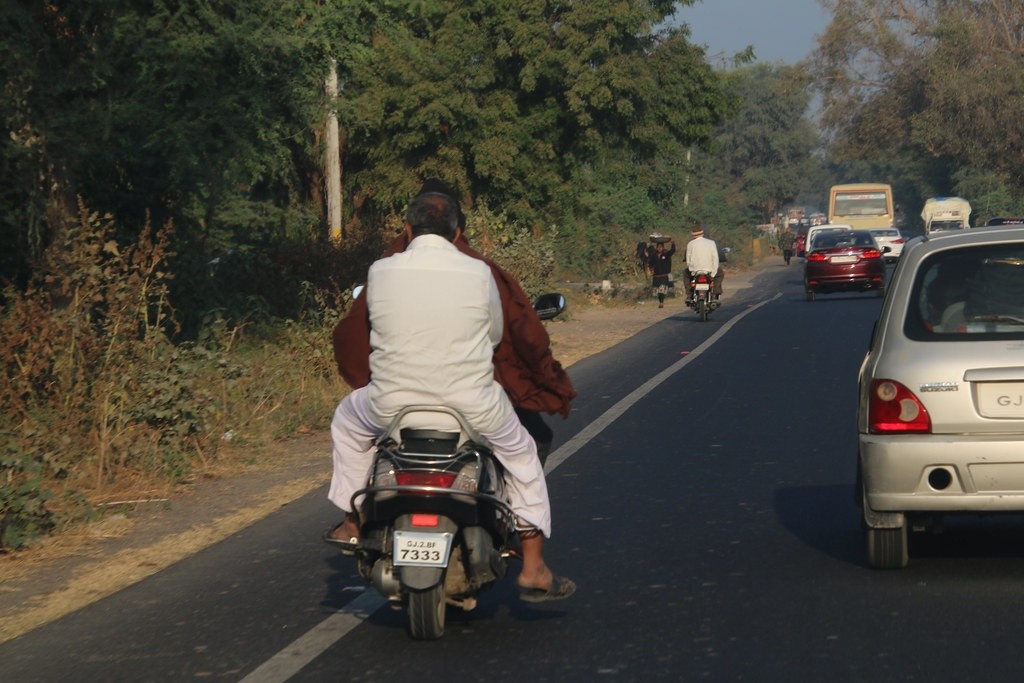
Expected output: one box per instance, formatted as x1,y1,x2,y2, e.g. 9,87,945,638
828,184,900,230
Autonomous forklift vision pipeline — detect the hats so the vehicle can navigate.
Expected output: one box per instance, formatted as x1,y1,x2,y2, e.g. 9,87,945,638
691,226,703,235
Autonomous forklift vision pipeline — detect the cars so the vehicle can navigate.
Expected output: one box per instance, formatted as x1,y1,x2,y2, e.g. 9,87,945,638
798,225,905,302
858,197,1024,570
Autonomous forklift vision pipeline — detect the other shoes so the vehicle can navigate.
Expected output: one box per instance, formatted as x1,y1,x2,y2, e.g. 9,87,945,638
659,304,663,307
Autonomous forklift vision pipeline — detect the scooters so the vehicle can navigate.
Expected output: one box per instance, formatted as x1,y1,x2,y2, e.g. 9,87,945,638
342,284,565,641
683,248,731,322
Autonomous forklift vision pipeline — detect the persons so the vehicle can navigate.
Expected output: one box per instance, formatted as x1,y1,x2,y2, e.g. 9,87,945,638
644,240,676,309
683,225,728,304
780,227,795,260
323,177,578,618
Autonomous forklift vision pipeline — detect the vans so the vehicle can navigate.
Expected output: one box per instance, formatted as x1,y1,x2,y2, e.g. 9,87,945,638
921,197,971,236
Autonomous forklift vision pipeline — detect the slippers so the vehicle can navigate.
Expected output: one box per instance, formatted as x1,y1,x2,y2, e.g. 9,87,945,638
323,528,358,548
521,576,578,603
